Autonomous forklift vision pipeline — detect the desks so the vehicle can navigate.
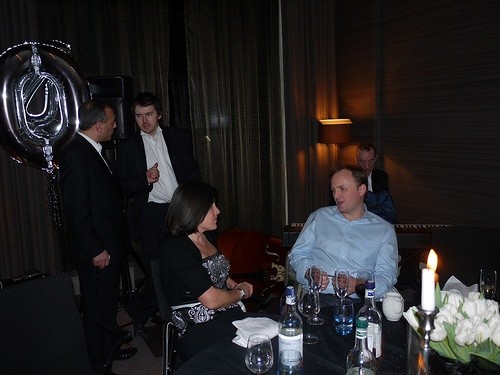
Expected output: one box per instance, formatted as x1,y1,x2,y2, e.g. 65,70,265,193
170,303,435,375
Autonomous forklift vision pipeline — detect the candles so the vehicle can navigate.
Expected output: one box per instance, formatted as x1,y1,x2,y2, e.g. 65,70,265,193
420,249,438,312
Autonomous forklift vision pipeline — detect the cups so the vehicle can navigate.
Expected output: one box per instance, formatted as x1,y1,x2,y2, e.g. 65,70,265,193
480,269,497,300
333,300,354,336
335,268,350,300
245,334,274,375
279,295,298,317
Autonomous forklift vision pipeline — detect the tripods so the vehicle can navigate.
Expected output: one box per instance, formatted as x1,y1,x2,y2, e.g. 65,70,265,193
110,137,150,309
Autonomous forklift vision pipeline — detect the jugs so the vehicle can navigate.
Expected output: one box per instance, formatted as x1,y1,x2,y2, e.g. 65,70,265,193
382,292,405,321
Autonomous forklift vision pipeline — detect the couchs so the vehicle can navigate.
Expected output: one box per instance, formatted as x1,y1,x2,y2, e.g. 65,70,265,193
216,229,292,295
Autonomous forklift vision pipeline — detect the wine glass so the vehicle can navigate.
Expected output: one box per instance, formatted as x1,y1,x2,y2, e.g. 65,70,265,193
355,270,375,311
298,264,325,344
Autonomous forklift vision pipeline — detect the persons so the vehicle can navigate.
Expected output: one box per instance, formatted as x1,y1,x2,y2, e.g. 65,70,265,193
113,93,205,324
155,183,253,349
287,165,399,326
354,142,400,230
51,99,160,360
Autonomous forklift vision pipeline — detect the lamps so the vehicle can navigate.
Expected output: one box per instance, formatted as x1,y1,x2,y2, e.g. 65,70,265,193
318,117,352,171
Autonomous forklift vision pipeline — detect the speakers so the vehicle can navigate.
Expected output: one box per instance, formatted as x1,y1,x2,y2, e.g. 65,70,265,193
85,73,133,140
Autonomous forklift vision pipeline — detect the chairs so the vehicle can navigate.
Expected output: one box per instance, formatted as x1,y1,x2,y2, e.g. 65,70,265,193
0,259,247,375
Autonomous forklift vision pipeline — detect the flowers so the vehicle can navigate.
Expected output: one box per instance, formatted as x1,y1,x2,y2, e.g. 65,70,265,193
403,275,500,367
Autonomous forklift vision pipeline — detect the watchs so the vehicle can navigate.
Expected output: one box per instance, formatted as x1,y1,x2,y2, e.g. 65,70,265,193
237,288,245,300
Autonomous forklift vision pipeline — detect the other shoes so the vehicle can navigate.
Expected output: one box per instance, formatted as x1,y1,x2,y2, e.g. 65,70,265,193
119,348,137,361
95,359,112,375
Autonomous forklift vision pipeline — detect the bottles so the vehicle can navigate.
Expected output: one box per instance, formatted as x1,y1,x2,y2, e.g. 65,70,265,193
346,316,378,375
277,286,303,375
356,280,383,370
